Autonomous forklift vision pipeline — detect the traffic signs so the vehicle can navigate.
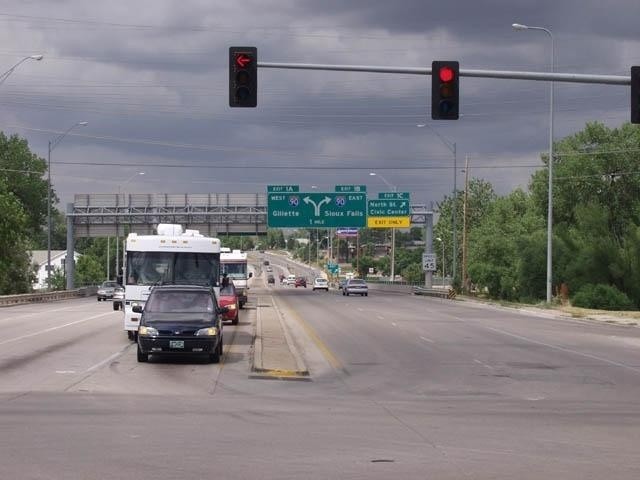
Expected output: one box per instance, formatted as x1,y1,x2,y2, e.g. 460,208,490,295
268,192,368,229
421,252,436,271
368,199,410,229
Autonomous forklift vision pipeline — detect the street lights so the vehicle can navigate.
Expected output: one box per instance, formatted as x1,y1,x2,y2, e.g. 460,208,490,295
117,171,144,280
436,237,445,288
417,122,459,287
312,185,324,191
369,171,399,281
513,22,557,302
0,53,45,92
47,121,89,295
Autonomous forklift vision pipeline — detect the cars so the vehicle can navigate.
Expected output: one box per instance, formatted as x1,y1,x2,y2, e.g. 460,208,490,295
98,280,123,300
134,285,230,367
114,289,126,310
220,277,241,326
260,248,370,296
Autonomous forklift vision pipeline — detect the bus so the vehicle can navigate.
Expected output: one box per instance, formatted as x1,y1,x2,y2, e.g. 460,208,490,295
220,247,253,308
122,219,230,347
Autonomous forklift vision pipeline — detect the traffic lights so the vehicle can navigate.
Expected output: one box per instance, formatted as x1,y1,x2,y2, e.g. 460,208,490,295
430,60,461,120
226,45,260,109
